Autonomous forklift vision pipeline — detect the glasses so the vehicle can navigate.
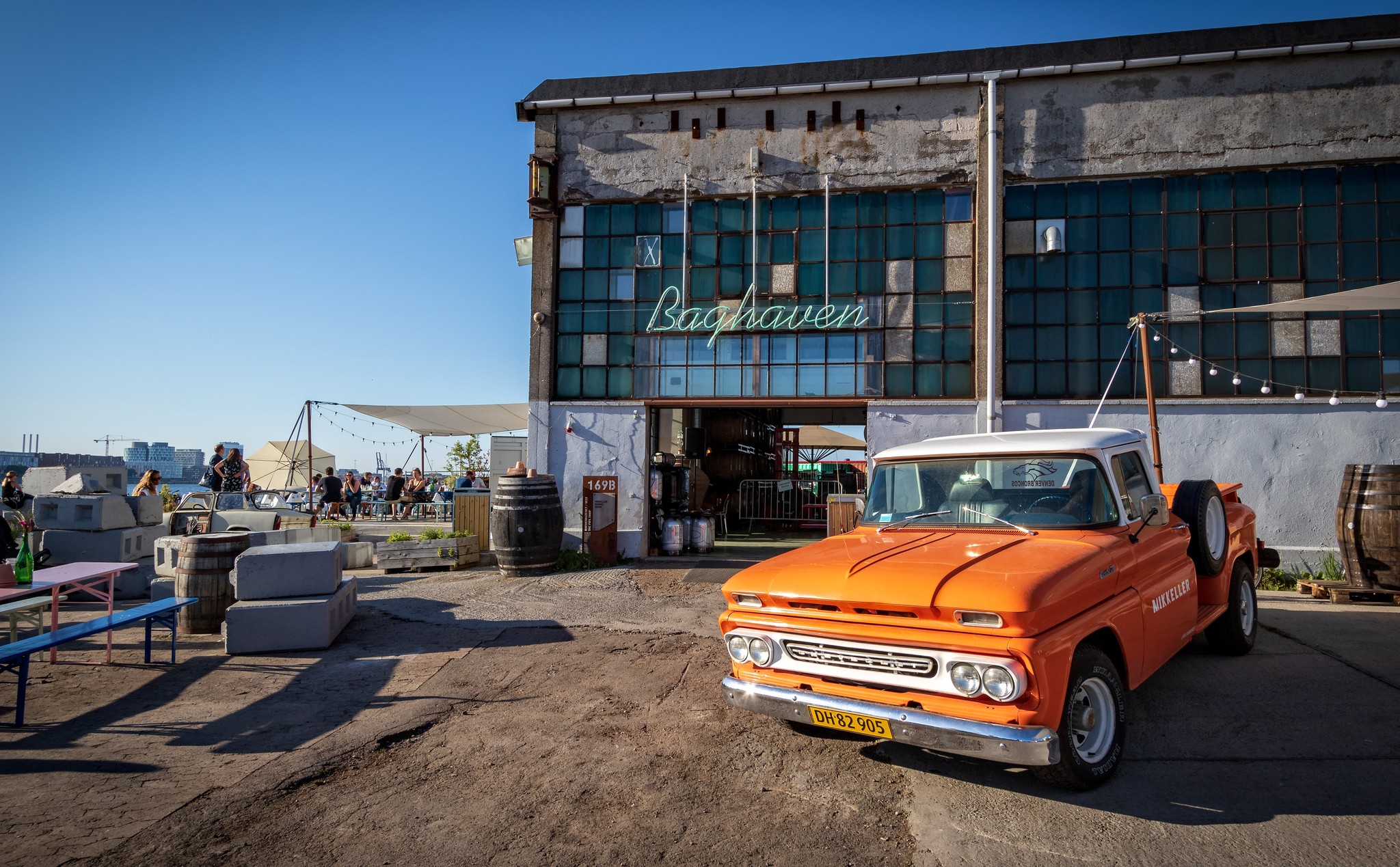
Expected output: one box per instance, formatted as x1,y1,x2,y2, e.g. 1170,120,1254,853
15,475,18,477
346,475,353,477
152,476,162,481
367,476,372,479
468,475,472,479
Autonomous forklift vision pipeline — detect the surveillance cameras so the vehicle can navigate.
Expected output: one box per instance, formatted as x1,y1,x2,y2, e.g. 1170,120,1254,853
533,312,545,324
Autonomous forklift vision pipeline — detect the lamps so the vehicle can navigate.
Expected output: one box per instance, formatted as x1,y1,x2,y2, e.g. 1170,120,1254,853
514,235,533,267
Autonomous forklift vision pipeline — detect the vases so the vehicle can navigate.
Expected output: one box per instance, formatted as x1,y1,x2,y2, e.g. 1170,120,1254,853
430,476,435,491
15,534,35,585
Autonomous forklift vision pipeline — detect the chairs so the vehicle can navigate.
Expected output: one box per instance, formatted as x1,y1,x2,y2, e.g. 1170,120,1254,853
937,476,1021,527
1057,468,1105,520
703,497,728,535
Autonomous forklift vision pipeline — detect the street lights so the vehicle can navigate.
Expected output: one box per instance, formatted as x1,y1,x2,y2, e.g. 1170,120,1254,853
430,460,434,474
354,460,356,470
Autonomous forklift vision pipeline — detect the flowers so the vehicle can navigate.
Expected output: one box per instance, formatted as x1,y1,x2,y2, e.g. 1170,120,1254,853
429,470,437,477
12,509,38,568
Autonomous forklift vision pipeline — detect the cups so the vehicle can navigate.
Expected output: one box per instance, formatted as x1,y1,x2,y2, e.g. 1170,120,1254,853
367,487,369,490
363,486,366,490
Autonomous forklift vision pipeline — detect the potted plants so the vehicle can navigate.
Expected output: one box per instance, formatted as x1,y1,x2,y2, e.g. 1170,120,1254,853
377,523,480,576
321,518,356,541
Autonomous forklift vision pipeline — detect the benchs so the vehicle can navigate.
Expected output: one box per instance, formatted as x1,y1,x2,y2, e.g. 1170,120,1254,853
284,500,453,522
0,596,68,661
800,521,828,528
0,597,199,727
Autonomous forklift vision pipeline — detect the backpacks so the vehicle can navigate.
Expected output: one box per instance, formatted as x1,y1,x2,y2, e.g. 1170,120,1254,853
423,493,435,503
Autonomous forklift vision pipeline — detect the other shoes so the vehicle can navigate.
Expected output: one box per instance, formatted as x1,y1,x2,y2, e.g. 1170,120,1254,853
360,513,368,516
368,512,376,516
350,517,356,521
346,515,349,521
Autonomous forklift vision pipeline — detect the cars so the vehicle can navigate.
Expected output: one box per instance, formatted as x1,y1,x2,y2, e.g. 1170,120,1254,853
717,425,1281,793
722,481,836,534
155,489,319,537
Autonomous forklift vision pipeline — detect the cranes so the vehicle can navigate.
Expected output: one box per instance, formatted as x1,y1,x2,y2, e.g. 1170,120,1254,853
93,435,140,455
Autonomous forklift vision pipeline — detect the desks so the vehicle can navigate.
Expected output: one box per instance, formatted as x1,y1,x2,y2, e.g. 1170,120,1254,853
376,490,455,522
804,504,827,507
300,490,374,518
0,562,139,665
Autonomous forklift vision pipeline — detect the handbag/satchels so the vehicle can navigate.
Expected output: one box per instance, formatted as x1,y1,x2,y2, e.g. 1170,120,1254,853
24,548,65,608
198,466,215,489
1,497,21,510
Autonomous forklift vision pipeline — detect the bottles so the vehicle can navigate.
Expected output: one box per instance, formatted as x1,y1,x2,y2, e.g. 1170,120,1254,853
427,486,430,492
369,484,372,490
15,533,34,585
430,478,435,492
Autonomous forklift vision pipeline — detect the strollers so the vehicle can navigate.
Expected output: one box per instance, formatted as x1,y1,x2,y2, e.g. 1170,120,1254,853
0,514,68,606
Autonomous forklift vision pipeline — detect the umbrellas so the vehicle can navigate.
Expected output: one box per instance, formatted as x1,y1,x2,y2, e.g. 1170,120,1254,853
782,426,867,493
245,440,336,499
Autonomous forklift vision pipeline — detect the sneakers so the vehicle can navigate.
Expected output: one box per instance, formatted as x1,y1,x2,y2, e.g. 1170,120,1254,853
400,516,410,521
392,517,399,521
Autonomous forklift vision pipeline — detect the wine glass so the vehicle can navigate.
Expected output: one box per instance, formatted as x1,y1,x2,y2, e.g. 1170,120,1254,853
375,482,379,490
366,484,369,490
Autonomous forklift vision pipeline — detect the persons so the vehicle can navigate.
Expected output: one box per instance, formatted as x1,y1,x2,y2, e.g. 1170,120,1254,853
209,444,226,492
214,448,249,492
239,455,249,492
306,465,486,520
244,476,267,491
131,469,161,496
2,471,35,508
0,515,19,564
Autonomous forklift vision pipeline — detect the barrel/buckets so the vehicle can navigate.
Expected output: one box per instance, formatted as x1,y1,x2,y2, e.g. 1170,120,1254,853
491,461,565,577
800,490,836,518
1335,463,1400,591
175,532,251,634
705,416,775,515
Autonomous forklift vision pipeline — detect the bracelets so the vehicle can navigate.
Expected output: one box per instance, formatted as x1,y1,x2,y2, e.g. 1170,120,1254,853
221,474,223,477
